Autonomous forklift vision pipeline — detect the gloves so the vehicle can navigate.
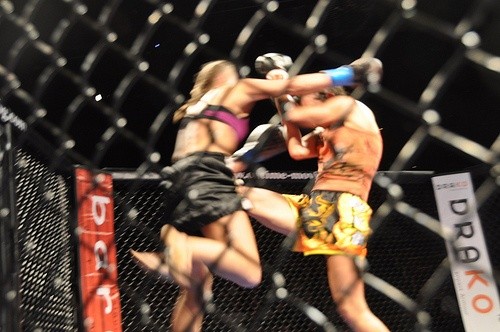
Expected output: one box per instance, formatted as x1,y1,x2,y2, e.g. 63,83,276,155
255,52,293,81
325,57,383,93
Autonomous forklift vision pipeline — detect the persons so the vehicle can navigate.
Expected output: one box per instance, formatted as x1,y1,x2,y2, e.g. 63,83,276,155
161,58,382,332
131,52,390,332
